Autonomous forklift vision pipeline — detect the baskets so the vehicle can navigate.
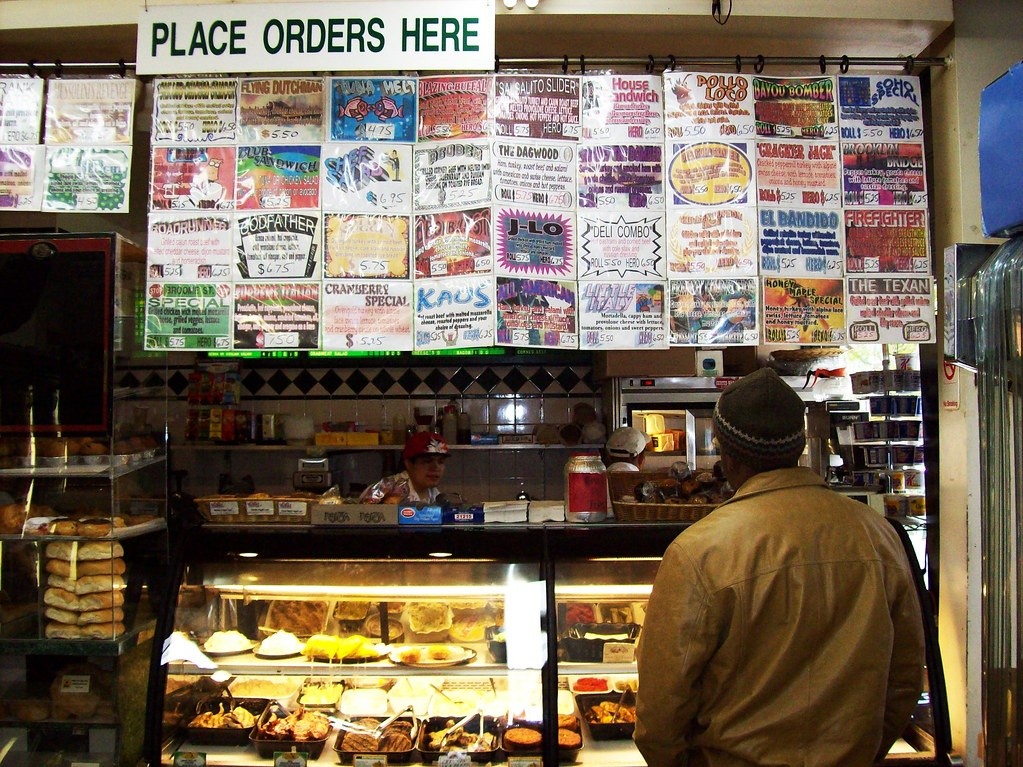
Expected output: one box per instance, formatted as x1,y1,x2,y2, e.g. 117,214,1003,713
608,464,714,506
611,497,723,523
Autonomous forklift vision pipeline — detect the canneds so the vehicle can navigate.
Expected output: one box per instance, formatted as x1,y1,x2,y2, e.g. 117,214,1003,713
563,451,609,524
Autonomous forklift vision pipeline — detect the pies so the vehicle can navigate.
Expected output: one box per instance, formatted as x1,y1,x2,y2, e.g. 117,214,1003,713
339,719,413,751
557,714,582,748
428,719,541,751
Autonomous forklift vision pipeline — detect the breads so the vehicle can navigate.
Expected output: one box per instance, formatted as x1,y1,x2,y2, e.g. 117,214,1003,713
635,460,731,504
0,426,164,720
401,644,451,662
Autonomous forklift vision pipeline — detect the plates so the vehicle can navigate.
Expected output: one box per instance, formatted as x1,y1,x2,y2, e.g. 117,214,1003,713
201,639,260,656
389,646,477,668
300,644,392,663
251,645,304,658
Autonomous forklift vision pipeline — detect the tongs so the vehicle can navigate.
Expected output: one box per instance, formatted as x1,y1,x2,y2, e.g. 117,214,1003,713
610,685,636,724
439,708,485,750
372,703,417,739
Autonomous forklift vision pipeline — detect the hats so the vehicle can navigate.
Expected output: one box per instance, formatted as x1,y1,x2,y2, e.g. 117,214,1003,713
713,367,806,455
606,427,652,457
403,430,451,461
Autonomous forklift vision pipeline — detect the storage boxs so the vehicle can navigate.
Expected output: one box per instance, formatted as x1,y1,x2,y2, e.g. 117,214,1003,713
315,431,346,447
345,432,379,445
398,505,459,524
312,503,399,524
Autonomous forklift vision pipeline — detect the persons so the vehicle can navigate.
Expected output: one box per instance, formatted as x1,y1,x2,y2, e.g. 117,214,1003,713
357,431,454,502
604,426,652,507
631,369,926,767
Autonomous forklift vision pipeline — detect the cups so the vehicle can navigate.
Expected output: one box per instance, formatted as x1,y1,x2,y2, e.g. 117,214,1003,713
415,425,430,433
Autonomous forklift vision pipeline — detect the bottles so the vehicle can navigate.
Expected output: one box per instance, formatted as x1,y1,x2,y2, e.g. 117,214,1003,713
436,397,471,443
563,450,607,523
392,408,404,441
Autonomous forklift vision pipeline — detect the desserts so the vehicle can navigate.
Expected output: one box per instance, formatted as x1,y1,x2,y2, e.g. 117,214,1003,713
205,627,381,658
